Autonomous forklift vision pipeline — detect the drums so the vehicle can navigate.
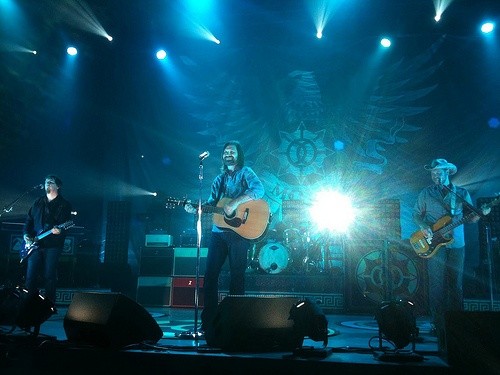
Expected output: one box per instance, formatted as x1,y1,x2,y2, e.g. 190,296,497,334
245,228,303,274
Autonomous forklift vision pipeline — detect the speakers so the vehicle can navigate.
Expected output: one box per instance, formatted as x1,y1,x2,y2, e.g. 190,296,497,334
139,247,174,276
63,293,163,349
172,278,204,307
346,252,384,314
388,250,431,314
174,248,208,277
437,310,500,367
136,277,172,307
206,295,305,352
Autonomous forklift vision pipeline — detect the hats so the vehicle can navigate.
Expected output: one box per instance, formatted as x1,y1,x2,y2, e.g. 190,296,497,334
424,158,458,176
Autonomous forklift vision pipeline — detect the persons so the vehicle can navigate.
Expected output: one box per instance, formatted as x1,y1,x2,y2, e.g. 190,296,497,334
22,175,71,314
412,159,492,337
183,141,264,339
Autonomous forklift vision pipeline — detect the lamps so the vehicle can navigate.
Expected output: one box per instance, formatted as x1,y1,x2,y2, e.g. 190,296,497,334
371,300,425,362
287,298,333,357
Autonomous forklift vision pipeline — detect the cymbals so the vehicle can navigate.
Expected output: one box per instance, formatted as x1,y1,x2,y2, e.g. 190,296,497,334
301,222,320,229
267,225,276,233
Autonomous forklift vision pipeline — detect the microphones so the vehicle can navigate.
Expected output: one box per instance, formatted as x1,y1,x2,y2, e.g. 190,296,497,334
199,151,210,160
26,184,44,194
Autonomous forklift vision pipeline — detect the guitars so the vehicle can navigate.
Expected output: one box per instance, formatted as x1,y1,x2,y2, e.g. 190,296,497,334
164,196,272,242
409,198,500,259
19,219,77,264
